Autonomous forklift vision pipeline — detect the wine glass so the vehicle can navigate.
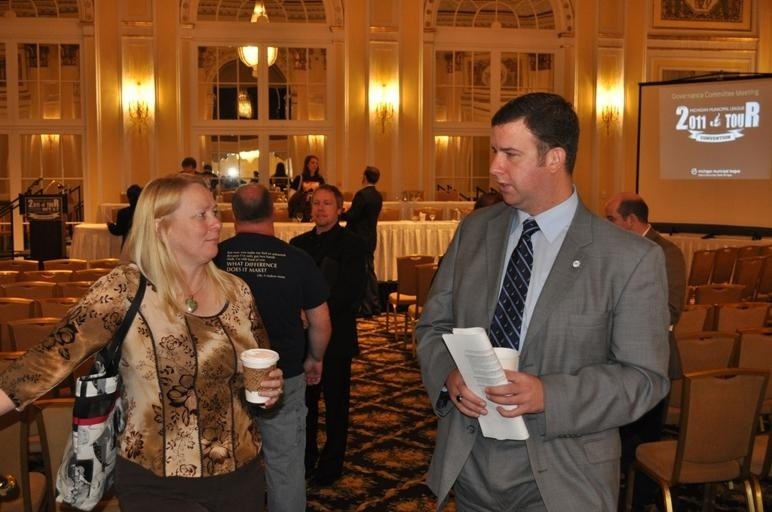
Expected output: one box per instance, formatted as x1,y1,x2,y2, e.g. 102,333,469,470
429,211,435,222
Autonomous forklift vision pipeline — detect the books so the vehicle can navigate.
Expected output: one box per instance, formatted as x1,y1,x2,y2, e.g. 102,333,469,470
440,322,531,444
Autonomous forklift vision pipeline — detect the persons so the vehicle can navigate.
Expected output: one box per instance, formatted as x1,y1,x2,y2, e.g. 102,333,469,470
104,156,326,252
343,165,386,318
409,90,676,510
1,171,285,511
288,185,370,488
211,181,333,511
602,192,685,442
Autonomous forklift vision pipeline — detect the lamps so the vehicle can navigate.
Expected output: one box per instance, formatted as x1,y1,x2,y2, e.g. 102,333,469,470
232,0,279,77
374,78,398,135
597,88,623,137
126,78,152,131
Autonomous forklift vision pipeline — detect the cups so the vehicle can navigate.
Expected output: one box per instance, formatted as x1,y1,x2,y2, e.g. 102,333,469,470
495,347,521,392
419,214,425,221
400,192,422,202
239,347,280,403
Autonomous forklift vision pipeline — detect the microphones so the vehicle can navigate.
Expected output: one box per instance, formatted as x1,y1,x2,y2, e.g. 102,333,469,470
33,176,43,185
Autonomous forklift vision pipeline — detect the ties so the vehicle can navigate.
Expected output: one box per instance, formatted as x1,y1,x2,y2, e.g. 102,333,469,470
490,220,540,349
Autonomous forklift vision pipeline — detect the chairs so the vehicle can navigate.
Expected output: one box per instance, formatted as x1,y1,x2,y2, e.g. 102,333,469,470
94,173,483,227
0,248,128,512
388,225,772,512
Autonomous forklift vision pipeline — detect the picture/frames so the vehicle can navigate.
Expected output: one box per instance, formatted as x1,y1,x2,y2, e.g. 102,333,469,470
651,0,753,34
460,50,534,98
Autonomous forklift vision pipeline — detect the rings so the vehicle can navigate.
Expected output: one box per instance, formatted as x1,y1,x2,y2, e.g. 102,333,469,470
455,393,464,403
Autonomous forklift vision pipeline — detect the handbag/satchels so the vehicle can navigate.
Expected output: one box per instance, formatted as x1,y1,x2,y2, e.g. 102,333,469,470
56,352,126,512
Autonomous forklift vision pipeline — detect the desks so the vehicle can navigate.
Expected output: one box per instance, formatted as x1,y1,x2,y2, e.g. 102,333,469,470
66,218,473,289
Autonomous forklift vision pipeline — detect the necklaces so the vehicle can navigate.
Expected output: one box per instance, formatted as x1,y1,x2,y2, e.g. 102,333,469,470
170,273,209,314
